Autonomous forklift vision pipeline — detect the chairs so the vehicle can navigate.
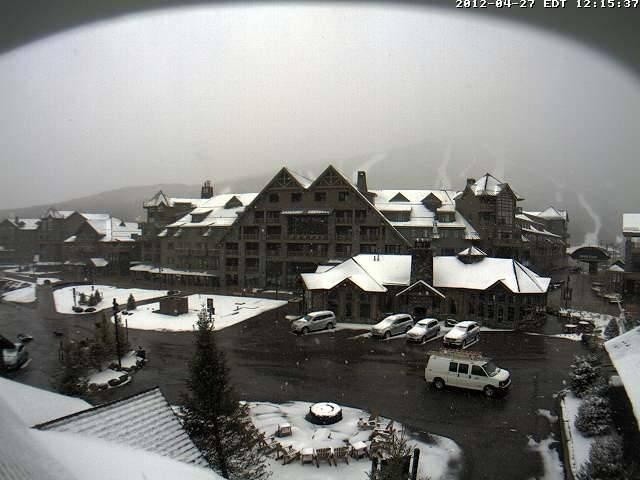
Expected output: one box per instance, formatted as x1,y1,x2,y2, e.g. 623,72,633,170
241,415,397,468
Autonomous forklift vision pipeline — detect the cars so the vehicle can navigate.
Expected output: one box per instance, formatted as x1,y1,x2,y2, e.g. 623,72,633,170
372,313,414,338
2,342,28,372
443,320,481,349
406,318,440,343
562,319,596,333
290,310,337,334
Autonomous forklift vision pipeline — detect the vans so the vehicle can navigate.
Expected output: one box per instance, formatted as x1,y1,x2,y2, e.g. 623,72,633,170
424,348,512,397
